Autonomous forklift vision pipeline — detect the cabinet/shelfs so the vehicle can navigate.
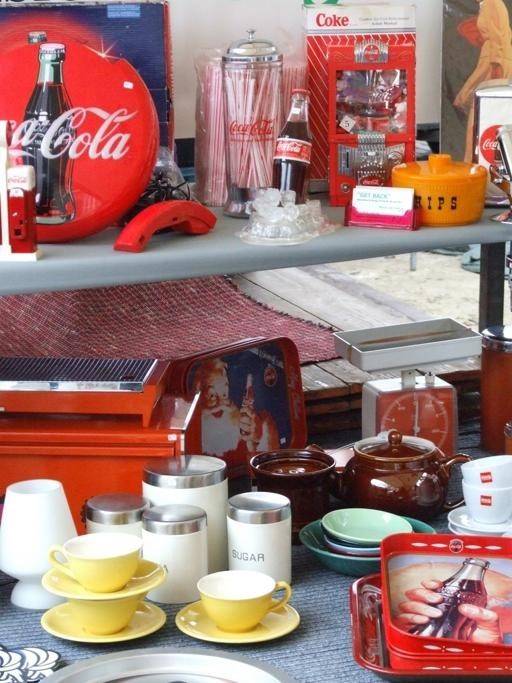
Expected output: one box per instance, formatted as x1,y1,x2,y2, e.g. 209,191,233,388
1,162,512,330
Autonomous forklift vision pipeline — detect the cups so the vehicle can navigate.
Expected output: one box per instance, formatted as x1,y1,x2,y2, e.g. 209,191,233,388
220,31,284,220
199,566,293,633
65,600,153,634
52,528,146,587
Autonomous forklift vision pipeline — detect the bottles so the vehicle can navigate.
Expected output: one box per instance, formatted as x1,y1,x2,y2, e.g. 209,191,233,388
21,41,79,226
140,455,229,575
140,502,210,604
410,553,491,638
28,30,48,42
272,86,312,200
492,128,507,175
238,374,258,439
226,491,295,589
82,493,150,540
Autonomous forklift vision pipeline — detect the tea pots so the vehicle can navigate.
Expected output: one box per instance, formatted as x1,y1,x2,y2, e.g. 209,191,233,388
330,428,470,516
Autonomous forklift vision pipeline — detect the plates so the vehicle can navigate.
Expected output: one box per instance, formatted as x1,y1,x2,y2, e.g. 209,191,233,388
41,561,168,602
173,335,309,480
174,598,305,643
347,530,512,681
36,646,297,683
39,602,170,647
443,506,512,537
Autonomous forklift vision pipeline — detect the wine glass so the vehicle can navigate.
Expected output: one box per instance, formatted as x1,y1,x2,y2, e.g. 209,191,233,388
0,478,78,611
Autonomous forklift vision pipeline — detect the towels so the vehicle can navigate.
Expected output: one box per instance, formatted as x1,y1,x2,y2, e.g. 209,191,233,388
0,273,343,367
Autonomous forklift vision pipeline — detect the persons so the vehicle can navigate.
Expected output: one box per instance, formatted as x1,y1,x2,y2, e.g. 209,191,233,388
396,580,503,645
192,358,280,467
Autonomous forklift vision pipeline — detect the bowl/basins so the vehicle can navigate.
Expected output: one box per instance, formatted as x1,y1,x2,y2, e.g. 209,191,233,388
249,447,338,511
459,450,510,522
294,501,435,575
393,153,490,225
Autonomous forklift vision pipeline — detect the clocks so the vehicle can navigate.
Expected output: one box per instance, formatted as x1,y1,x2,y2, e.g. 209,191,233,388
361,369,459,458
